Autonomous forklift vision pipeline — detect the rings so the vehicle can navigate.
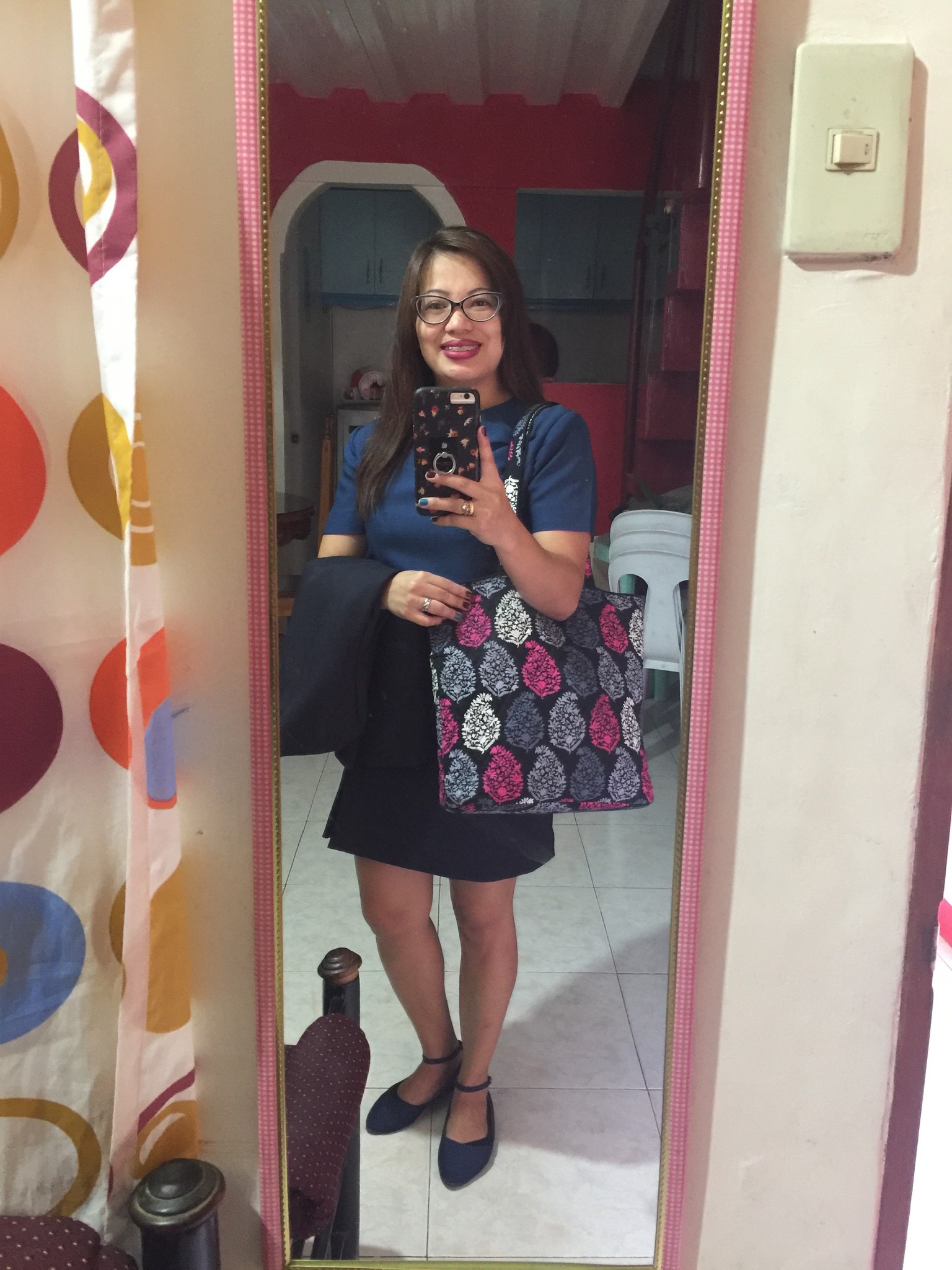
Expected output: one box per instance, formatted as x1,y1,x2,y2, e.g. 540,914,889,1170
422,598,431,612
462,500,474,515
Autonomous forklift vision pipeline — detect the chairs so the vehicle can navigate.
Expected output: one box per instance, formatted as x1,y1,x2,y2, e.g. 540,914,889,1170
608,508,694,722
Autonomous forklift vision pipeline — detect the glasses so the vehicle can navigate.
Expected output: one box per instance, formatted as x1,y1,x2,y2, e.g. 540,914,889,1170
412,293,511,326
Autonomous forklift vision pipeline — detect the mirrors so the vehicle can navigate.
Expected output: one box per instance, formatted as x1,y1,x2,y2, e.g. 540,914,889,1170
233,0,753,1270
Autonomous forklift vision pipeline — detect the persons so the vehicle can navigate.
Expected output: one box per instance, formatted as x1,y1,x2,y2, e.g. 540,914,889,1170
317,226,599,1188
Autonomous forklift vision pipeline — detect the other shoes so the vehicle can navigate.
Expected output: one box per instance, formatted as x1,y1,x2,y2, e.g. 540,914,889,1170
365,1041,462,1135
440,1075,498,1192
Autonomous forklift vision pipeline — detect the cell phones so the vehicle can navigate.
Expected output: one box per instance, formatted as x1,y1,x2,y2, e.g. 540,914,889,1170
413,386,481,517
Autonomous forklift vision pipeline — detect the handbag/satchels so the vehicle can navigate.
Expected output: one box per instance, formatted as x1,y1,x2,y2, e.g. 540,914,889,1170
426,398,652,816
